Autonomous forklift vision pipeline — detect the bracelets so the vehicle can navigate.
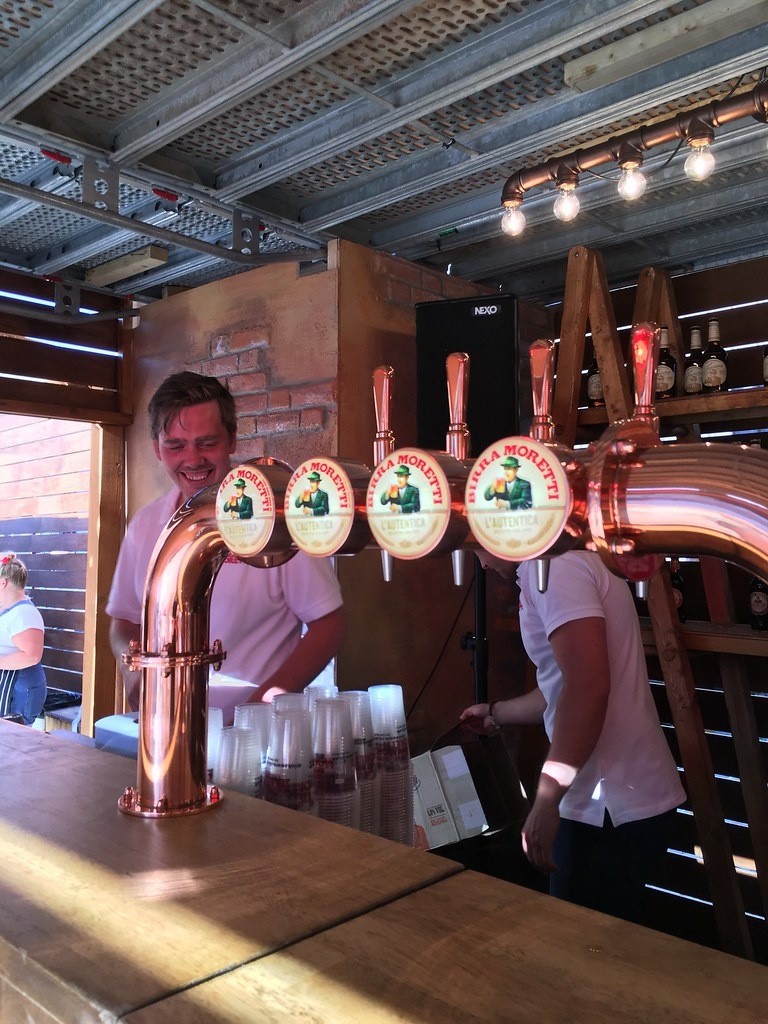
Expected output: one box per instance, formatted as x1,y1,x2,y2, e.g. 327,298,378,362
488,701,503,730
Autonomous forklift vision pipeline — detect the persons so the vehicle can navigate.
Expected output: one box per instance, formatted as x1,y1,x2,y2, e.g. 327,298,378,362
104,370,346,726
459,550,687,922
0,551,47,726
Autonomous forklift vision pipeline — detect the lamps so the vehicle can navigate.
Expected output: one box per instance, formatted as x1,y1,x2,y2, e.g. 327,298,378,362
501,82,768,239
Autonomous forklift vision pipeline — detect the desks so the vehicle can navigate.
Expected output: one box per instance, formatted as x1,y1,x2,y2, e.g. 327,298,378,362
0,715,768,1024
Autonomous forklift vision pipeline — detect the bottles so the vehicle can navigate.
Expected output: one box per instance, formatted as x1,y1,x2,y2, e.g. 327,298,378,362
762,346,768,386
731,439,761,449
655,324,677,400
701,317,730,393
683,325,705,395
669,557,686,624
587,350,605,408
749,576,768,631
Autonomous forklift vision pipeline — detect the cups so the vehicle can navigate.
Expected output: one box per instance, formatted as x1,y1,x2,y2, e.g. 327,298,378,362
207,685,417,851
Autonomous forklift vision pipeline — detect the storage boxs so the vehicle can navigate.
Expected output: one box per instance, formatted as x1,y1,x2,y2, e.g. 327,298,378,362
410,716,531,852
